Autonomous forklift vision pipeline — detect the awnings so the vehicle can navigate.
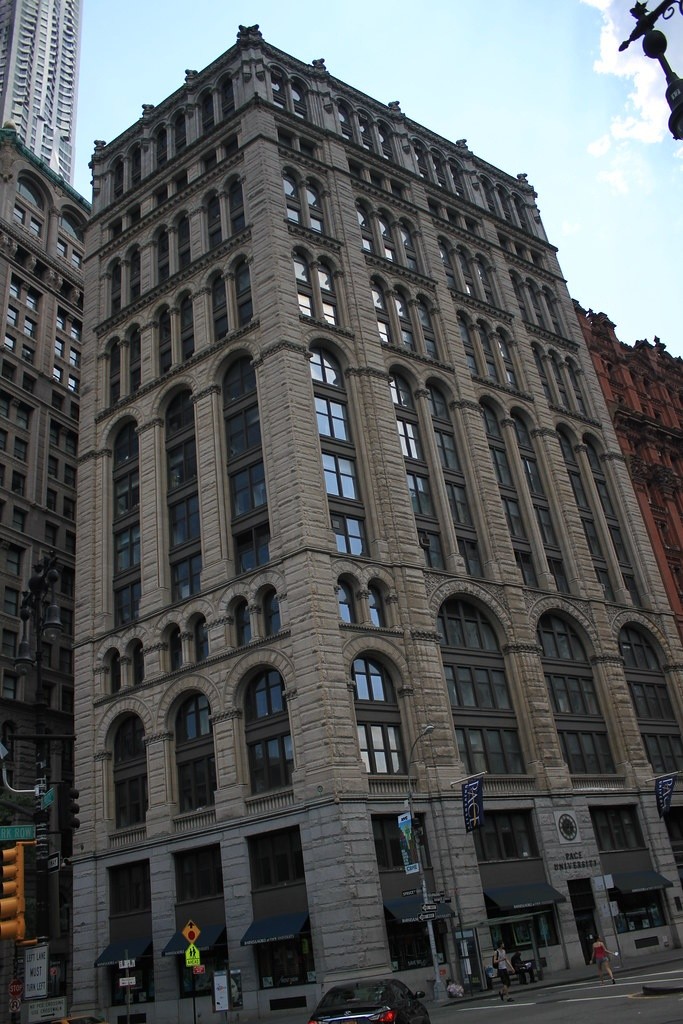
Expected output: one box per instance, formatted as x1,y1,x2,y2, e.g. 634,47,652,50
609,871,674,894
383,896,455,925
162,924,226,957
482,883,567,911
240,912,310,946
94,938,154,968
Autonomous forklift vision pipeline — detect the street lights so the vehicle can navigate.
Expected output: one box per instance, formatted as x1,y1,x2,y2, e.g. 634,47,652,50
408,726,449,1003
57,784,80,837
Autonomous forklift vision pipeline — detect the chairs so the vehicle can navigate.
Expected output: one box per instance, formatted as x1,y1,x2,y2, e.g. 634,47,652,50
343,991,355,1002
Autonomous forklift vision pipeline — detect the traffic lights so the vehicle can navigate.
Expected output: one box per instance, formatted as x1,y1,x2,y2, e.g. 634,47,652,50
0,846,24,941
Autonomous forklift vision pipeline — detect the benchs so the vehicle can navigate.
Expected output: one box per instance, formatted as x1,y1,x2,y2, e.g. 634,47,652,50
487,969,526,989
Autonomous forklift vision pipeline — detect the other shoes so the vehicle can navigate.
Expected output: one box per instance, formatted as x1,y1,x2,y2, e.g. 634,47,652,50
507,998,514,1002
612,979,615,984
520,982,528,985
530,980,537,983
499,991,503,1000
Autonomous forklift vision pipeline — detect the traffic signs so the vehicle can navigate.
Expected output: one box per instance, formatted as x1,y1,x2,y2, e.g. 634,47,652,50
421,904,437,911
417,913,436,922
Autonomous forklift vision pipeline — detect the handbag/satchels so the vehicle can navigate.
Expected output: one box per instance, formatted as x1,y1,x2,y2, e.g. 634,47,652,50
493,951,499,969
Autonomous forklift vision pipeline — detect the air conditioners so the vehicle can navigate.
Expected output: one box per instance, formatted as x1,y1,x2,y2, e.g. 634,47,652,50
420,538,431,547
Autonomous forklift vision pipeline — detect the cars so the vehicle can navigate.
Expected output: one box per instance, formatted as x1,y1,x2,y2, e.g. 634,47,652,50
52,1014,109,1024
307,979,432,1024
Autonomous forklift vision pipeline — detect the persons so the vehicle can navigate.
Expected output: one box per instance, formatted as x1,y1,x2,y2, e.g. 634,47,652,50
492,940,515,1002
590,935,619,985
511,952,537,985
